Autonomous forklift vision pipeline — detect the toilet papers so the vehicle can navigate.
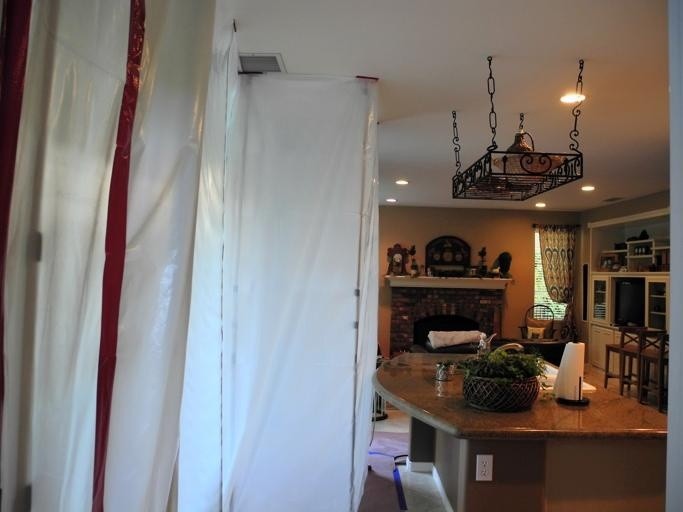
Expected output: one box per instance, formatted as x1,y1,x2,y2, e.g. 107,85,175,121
551,342,585,400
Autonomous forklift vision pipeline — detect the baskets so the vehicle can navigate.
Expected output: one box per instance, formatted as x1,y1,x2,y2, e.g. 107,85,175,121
461,368,540,414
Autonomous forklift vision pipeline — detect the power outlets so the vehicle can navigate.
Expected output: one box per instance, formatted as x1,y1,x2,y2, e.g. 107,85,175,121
476,451,492,482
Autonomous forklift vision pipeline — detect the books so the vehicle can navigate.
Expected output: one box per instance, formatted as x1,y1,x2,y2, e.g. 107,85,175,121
538,363,597,391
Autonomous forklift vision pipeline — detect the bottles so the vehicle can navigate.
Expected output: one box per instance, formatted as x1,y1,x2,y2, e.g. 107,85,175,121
410,259,419,278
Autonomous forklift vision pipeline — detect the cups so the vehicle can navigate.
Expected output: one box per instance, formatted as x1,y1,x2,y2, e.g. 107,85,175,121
435,362,449,381
468,268,478,275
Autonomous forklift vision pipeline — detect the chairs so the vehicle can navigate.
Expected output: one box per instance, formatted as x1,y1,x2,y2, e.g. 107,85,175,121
516,304,557,338
604,325,669,413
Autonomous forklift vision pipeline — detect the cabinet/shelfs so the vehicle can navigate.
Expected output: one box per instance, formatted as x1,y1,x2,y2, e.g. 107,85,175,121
586,206,670,389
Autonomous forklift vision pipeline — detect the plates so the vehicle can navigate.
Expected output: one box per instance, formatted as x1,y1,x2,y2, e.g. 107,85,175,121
464,274,481,278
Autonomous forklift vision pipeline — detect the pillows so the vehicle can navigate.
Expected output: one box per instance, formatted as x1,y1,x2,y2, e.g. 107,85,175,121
526,317,552,341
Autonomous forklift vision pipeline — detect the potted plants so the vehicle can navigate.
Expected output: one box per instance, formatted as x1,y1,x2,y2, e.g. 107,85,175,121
456,346,545,414
